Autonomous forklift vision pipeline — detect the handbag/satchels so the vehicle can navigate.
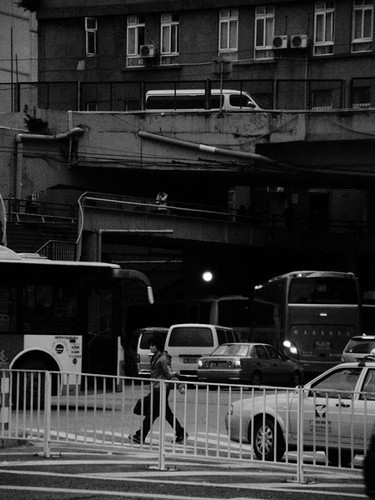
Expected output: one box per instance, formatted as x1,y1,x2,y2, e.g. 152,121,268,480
134,392,151,416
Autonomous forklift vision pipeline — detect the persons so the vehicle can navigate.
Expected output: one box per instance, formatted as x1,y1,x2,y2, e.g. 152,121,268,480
156,189,167,213
127,338,189,444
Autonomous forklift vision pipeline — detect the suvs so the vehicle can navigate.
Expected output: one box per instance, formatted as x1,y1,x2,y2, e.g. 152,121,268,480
340,333,375,363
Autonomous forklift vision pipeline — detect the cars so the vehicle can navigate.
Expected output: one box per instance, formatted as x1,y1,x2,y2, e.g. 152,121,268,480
197,343,304,392
225,361,375,465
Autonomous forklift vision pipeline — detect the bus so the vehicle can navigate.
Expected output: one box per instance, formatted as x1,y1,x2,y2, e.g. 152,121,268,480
189,295,249,341
0,245,154,409
247,271,362,383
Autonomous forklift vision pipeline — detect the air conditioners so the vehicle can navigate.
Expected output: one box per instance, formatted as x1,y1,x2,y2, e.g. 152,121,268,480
272,35,288,49
140,44,156,58
291,34,308,48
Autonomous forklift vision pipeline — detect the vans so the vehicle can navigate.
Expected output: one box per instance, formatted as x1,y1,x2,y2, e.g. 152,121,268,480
144,89,262,111
162,323,241,388
135,328,168,384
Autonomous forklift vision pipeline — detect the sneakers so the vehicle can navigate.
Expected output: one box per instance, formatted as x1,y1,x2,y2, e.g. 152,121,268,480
128,435,145,444
172,432,188,444
